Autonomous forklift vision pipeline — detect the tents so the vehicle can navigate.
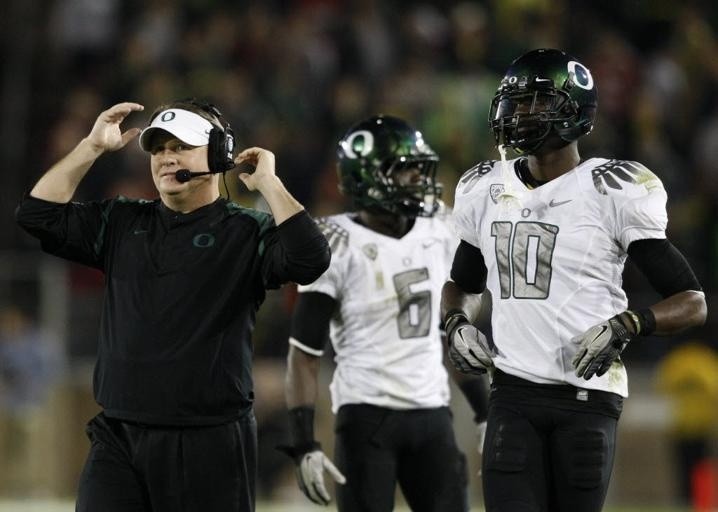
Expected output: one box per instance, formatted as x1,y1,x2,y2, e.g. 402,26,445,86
149,104,235,172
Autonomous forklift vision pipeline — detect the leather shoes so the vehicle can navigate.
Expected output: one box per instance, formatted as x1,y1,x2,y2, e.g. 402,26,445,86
567,315,632,384
448,320,497,378
292,450,349,507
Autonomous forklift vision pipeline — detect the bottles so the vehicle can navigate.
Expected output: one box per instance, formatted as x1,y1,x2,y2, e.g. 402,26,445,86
138,108,214,154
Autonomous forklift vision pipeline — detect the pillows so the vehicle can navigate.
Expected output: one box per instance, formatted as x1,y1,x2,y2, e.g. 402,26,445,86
335,111,441,220
487,47,598,158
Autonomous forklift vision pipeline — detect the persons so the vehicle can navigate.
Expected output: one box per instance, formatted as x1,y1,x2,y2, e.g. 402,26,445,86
1,0,717,512
286,114,491,510
9,98,332,510
440,49,708,510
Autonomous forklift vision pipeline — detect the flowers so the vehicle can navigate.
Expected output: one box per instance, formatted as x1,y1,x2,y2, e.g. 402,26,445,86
174,168,221,182
